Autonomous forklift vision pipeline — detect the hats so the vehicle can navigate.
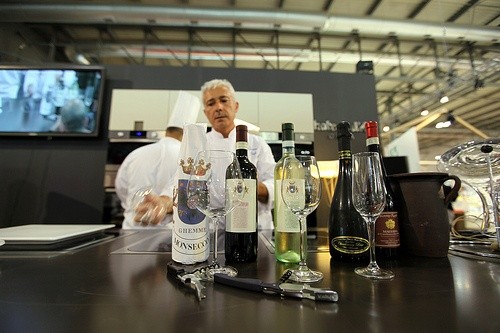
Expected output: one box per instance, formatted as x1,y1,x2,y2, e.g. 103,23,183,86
167,90,200,130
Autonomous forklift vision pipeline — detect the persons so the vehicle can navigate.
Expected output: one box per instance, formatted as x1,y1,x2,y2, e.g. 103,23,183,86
134,79,281,230
0,70,92,132
114,91,201,230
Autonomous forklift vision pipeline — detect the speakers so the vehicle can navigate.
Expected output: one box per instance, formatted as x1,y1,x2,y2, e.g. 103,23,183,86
383,157,408,175
356,61,373,74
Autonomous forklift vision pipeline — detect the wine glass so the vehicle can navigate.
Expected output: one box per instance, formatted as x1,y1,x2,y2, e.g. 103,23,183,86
186,150,238,282
352,152,395,280
280,156,324,282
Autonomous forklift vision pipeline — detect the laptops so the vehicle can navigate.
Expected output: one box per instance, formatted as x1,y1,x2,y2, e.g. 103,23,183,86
0,224,116,251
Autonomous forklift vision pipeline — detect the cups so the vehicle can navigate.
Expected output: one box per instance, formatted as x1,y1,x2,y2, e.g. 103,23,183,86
385,172,461,258
130,185,166,226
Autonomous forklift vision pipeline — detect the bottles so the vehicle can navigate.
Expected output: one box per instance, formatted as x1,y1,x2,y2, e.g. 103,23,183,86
328,121,370,265
274,122,308,262
360,120,403,268
225,125,258,265
171,124,209,265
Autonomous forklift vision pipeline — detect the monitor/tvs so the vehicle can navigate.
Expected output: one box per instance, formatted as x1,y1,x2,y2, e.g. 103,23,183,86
0,64,107,138
